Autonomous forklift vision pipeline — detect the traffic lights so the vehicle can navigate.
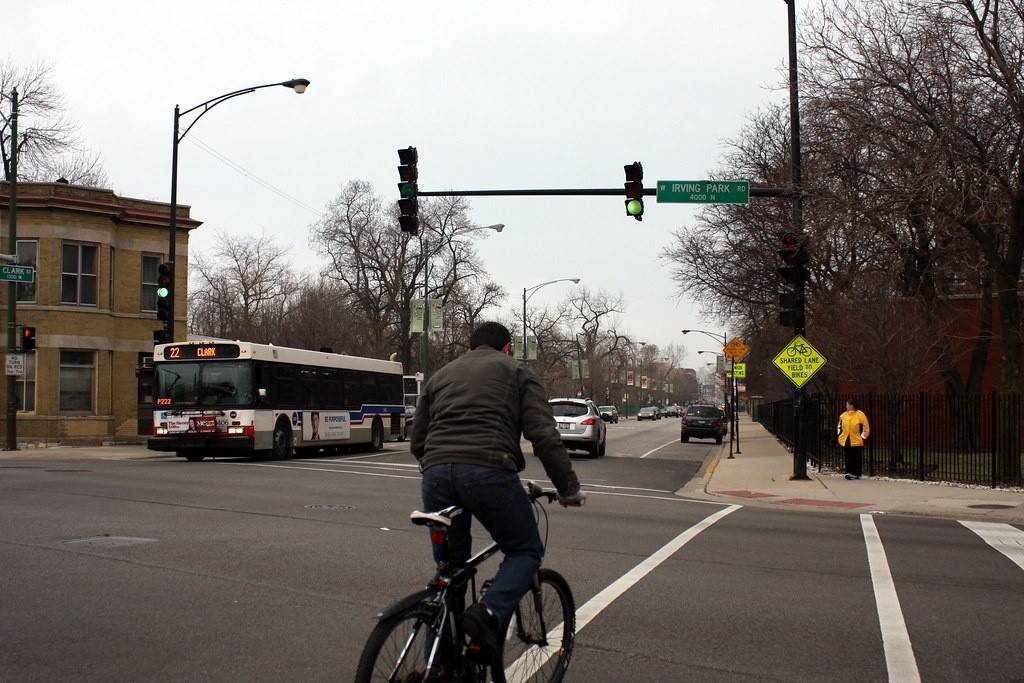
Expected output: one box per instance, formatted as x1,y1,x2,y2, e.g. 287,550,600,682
156,260,176,322
776,232,806,328
153,327,172,346
624,161,644,221
20,326,36,354
397,146,420,236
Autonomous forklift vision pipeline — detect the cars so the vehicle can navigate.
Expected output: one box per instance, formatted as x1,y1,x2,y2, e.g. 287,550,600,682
665,406,679,419
652,405,688,420
405,405,416,426
637,406,657,421
718,407,731,436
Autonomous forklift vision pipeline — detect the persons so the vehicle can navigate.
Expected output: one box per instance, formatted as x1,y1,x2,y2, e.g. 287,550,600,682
311,412,320,439
408,321,586,683
189,417,196,430
836,400,870,480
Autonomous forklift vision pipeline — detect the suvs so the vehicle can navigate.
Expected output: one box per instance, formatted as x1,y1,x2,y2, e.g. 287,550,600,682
533,396,610,459
681,404,728,445
597,405,619,424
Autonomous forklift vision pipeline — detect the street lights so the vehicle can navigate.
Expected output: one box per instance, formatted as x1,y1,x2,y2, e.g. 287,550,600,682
681,329,735,459
639,357,669,408
423,224,506,386
166,78,312,342
698,350,728,415
524,278,581,367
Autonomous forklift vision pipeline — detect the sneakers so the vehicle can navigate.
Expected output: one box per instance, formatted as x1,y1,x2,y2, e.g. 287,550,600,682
459,601,504,667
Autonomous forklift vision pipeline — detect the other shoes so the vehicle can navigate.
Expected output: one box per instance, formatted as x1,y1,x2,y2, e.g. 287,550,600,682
845,475,859,479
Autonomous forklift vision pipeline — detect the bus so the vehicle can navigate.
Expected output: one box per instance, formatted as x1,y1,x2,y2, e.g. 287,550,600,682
135,340,407,462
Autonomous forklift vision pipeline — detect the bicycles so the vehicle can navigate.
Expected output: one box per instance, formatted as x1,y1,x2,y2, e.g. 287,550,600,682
354,478,586,683
